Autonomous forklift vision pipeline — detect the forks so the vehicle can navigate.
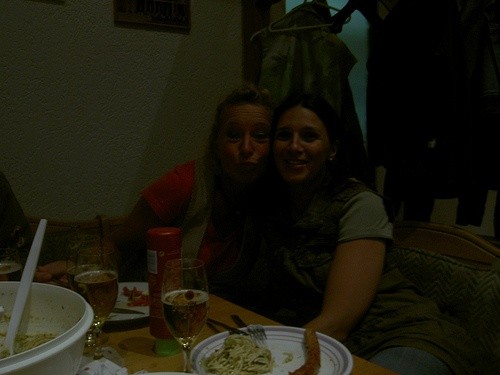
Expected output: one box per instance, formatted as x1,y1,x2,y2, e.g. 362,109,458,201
231,314,267,341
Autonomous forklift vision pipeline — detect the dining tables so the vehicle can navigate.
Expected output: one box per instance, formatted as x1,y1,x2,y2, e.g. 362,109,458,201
78,288,402,375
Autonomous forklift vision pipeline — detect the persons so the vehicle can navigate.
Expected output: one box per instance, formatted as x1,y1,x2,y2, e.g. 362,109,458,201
35,81,481,375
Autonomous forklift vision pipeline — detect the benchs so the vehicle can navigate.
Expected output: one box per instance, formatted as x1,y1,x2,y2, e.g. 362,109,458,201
381,223,500,375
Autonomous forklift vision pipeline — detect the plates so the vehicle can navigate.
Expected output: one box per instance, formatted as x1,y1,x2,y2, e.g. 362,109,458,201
189,324,354,375
108,282,150,321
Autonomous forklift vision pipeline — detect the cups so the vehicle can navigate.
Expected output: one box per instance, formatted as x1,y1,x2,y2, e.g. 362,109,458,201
76,247,119,347
66,237,102,294
0,248,23,281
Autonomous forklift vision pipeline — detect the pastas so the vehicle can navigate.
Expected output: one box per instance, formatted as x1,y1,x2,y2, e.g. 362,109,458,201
0,332,56,359
203,333,273,375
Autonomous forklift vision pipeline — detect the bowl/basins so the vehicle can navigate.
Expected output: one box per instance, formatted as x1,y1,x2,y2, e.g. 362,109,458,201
0,279,94,375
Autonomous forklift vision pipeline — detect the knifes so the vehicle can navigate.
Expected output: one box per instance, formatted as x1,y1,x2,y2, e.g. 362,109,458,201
207,318,249,337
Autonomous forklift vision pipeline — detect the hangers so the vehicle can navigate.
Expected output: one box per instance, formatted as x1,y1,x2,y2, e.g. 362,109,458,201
250,0,351,41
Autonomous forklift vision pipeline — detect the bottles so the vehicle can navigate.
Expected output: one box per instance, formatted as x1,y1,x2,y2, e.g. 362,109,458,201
146,228,184,340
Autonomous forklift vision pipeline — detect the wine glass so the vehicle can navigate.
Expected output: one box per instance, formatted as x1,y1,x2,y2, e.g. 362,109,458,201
161,257,209,375
